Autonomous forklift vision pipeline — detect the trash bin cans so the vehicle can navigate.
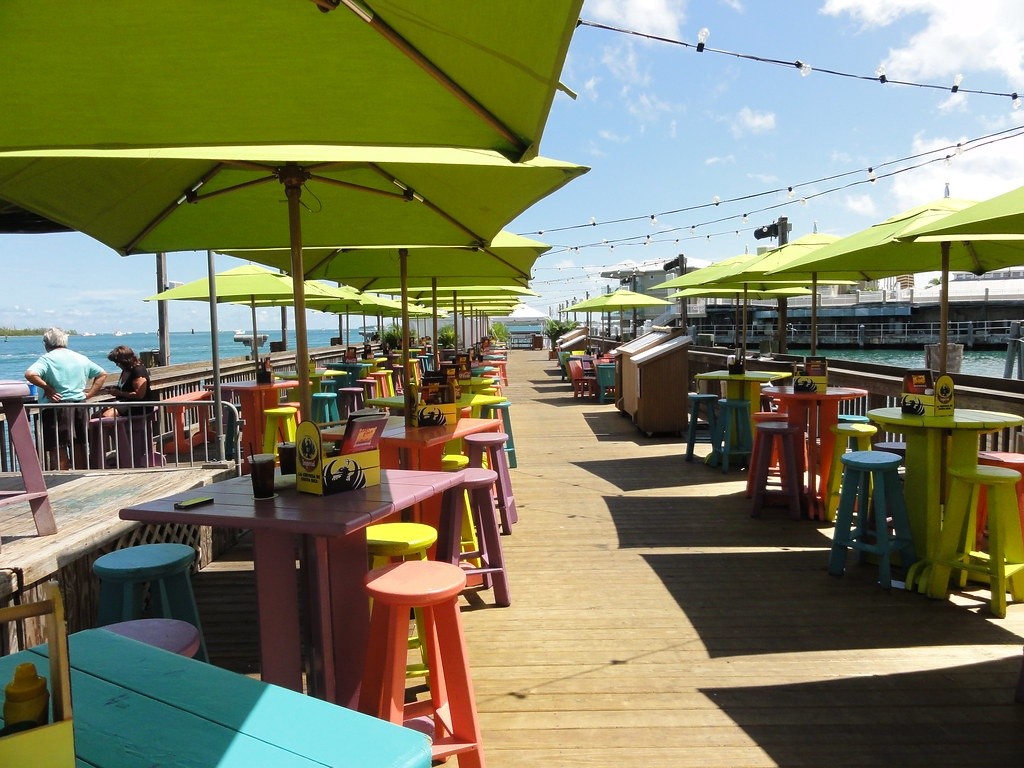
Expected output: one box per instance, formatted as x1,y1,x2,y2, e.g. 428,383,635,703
532,336,543,350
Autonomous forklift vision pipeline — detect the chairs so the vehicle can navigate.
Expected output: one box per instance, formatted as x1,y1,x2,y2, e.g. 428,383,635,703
556,349,616,404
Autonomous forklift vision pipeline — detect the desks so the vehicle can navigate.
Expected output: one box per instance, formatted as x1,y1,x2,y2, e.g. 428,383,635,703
583,357,595,361
0,342,509,768
694,370,792,465
761,386,869,520
866,406,1024,595
599,356,615,363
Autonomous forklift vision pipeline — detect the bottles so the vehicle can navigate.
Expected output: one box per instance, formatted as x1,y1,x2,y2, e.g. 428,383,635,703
275,442,297,475
248,453,278,500
3,664,50,729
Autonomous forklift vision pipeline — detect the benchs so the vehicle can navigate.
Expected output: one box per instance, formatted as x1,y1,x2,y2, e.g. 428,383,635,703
88,404,168,468
161,389,218,455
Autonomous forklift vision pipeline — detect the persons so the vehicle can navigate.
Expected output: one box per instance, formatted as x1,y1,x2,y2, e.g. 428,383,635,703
104,346,152,468
26,327,107,472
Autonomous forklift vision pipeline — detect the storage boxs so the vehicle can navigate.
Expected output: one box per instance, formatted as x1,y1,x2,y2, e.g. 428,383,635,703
294,419,381,495
409,383,457,427
422,374,461,399
901,374,955,418
458,368,470,386
256,357,276,384
362,344,375,360
792,360,828,393
342,347,357,364
727,355,745,374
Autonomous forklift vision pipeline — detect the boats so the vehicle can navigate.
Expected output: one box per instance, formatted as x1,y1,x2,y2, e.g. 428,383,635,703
81,333,96,336
233,328,245,335
126,331,132,334
359,325,388,337
4,339,8,342
599,254,1024,328
113,331,126,336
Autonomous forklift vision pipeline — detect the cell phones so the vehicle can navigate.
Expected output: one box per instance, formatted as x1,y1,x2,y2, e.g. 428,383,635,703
174,497,214,509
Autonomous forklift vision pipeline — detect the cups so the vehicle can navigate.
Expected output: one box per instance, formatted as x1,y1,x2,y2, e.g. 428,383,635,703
26,381,37,396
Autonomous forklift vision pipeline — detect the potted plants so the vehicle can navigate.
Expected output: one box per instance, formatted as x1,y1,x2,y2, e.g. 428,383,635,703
545,317,579,360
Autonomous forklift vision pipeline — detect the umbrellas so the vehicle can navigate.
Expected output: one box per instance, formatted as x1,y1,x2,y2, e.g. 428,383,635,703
559,290,676,354
647,185,1024,378
0,0,589,423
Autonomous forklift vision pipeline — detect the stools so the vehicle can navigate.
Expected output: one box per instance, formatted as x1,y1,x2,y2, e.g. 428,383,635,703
686,394,1024,618
93,542,210,664
99,618,200,658
262,353,518,768
0,380,59,538
